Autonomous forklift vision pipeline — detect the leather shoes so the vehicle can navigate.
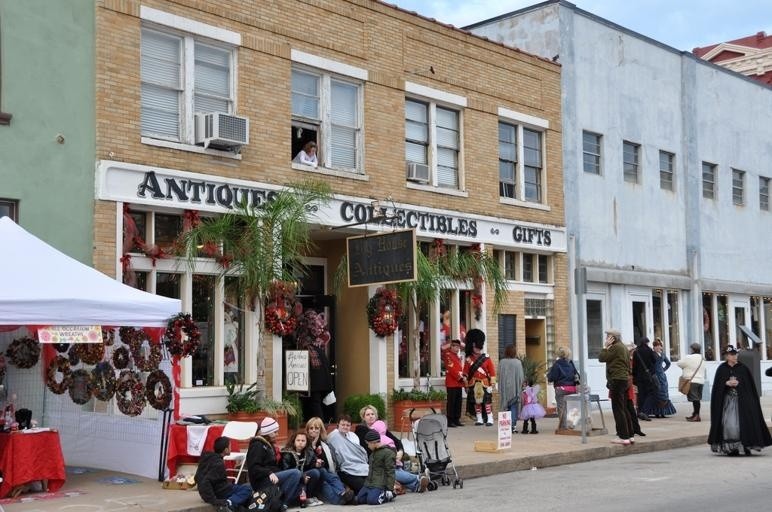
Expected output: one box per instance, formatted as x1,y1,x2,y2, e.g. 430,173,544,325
686,414,700,421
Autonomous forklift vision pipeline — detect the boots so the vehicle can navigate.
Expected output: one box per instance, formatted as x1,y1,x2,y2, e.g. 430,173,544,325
486,413,494,426
475,413,484,425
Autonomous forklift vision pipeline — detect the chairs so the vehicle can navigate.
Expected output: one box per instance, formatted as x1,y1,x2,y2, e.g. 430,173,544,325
217,421,258,484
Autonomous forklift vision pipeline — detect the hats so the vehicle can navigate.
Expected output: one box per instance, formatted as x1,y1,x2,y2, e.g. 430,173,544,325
260,416,280,436
605,329,621,336
451,339,460,346
721,345,738,354
365,430,380,441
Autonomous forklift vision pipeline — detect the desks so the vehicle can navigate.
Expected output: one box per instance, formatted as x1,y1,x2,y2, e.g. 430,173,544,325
166,422,240,480
0,427,66,499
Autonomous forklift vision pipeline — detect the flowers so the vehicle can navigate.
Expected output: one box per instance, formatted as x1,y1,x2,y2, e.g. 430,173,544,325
5,311,202,416
366,287,405,338
264,279,331,347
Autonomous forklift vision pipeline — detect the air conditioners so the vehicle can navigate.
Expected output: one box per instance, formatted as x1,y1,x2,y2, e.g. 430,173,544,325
197,112,250,150
407,163,430,181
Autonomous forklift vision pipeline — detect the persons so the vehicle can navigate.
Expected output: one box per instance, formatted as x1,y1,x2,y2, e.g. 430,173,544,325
444,339,580,434
194,405,429,512
599,328,706,445
707,344,772,457
292,141,318,169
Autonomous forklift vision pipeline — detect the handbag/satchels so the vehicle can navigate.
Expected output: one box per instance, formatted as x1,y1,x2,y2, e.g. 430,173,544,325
652,374,660,386
574,374,580,385
678,377,691,395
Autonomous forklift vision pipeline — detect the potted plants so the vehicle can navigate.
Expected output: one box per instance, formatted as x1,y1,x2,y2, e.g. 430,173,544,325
391,386,447,432
223,372,299,451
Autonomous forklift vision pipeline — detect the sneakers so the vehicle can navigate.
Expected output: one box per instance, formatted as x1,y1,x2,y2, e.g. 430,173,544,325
417,467,431,492
610,436,634,444
307,496,323,506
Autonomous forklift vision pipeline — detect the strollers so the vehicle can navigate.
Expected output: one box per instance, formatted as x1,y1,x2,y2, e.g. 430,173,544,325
409,406,463,492
